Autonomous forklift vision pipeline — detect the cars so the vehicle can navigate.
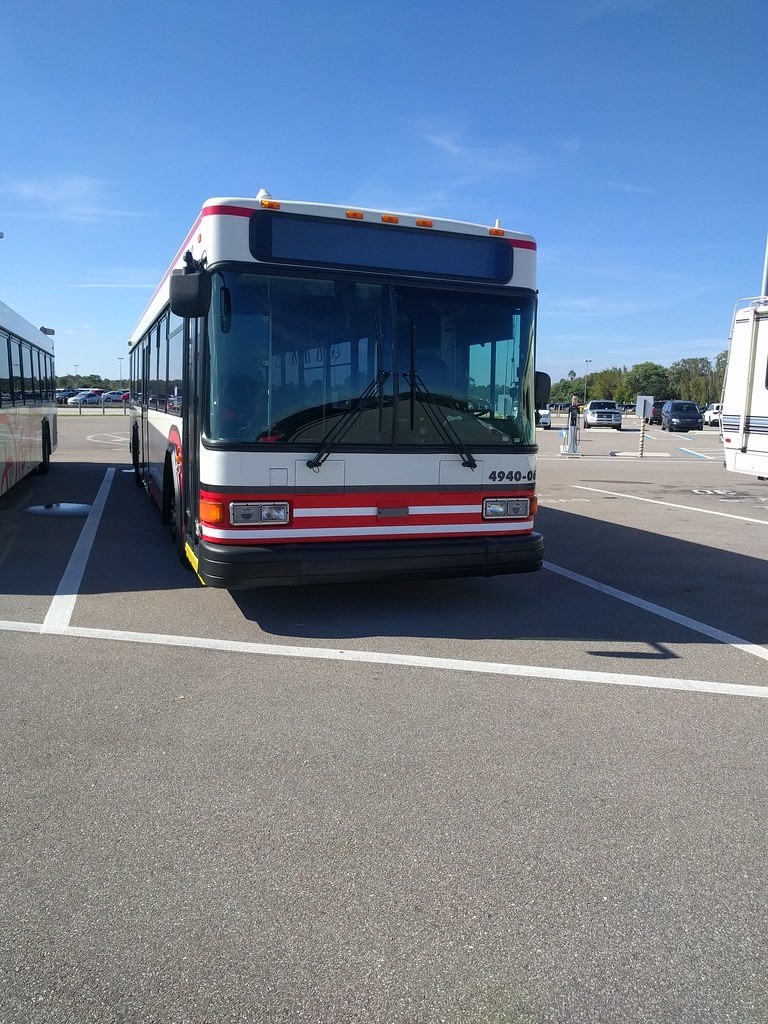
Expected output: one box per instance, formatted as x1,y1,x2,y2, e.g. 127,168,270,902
3,390,45,400
703,403,723,426
55,387,130,405
130,391,182,412
661,399,705,434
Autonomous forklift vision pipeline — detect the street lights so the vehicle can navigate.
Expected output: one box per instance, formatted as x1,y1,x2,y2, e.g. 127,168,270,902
118,357,124,389
582,358,593,404
73,365,80,389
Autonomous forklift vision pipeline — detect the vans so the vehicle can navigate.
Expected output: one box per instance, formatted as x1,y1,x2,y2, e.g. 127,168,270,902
532,409,552,429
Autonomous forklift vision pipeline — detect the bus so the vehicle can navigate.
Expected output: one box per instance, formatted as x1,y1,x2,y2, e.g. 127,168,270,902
0,296,60,498
120,190,551,588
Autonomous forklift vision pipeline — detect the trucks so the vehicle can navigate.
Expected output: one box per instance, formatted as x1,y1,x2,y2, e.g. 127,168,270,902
637,394,669,424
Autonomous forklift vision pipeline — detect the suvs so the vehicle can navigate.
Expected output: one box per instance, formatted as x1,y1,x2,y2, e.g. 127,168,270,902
585,396,622,431
